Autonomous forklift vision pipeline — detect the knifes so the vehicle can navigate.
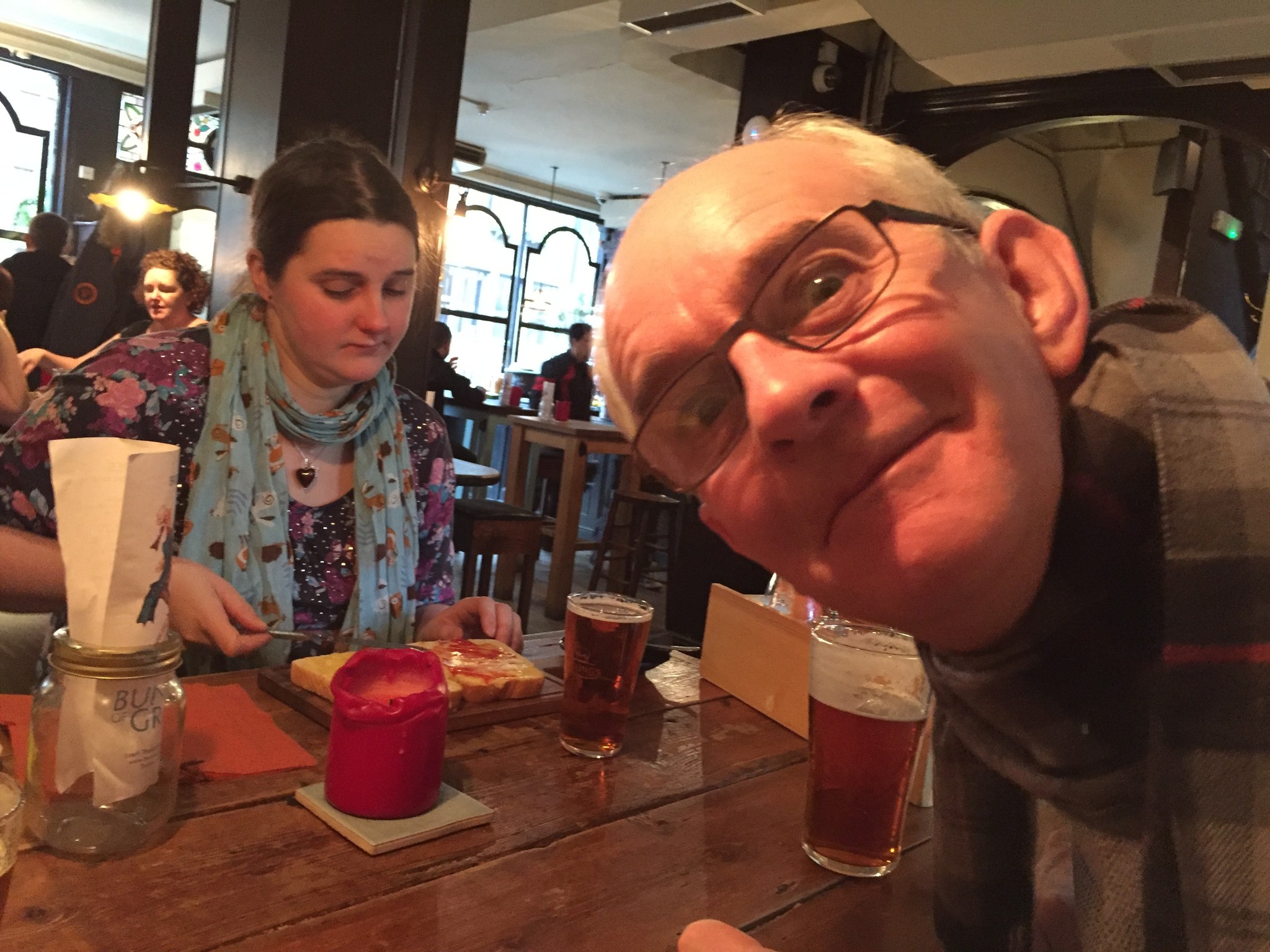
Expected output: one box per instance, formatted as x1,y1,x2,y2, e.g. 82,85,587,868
239,630,430,652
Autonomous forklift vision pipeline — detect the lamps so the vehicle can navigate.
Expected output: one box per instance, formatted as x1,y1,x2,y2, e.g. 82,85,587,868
91,160,256,225
445,181,473,240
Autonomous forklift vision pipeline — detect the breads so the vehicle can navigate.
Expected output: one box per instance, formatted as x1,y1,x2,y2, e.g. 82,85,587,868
290,650,462,715
407,638,547,702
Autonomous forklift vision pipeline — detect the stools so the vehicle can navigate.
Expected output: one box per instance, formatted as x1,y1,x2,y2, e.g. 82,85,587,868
447,503,542,637
446,456,504,505
586,483,680,635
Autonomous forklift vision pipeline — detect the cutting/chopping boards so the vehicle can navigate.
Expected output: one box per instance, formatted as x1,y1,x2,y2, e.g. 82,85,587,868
256,666,568,733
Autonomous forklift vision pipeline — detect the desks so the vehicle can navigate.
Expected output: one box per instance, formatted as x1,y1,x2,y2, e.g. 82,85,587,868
2,628,967,952
434,386,545,506
505,413,640,621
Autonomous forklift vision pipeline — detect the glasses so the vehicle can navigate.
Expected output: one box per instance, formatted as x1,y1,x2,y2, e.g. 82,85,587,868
631,199,981,493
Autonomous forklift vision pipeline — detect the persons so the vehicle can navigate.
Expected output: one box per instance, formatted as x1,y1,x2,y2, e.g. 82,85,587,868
0,140,522,664
426,321,486,466
0,187,212,424
600,113,1270,952
530,323,596,521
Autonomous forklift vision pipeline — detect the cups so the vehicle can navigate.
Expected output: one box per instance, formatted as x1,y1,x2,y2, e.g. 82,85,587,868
0,770,24,925
558,590,654,759
509,386,523,407
555,400,571,421
797,621,932,878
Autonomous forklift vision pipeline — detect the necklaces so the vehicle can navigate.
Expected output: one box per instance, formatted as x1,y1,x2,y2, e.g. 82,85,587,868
285,433,332,489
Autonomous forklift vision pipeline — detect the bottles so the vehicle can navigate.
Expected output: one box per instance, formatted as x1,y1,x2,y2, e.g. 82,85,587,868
24,631,186,856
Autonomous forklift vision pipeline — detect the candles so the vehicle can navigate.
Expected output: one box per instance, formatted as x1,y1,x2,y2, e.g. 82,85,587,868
323,647,442,822
